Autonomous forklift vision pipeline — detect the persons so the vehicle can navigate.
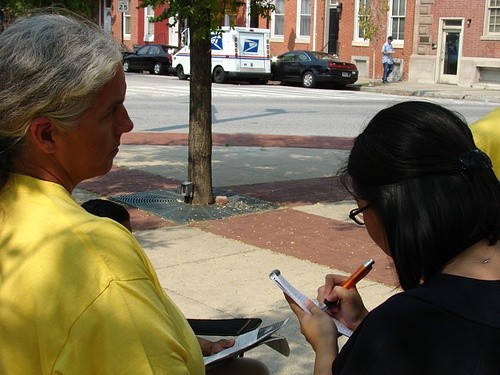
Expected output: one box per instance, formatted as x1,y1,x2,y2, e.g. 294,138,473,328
0,15,236,375
382,36,396,83
81,198,269,375
282,101,500,375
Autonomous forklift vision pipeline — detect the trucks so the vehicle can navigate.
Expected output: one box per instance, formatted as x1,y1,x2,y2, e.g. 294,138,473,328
171,25,271,85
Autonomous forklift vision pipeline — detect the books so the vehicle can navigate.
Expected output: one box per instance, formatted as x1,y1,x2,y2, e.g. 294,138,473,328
269,269,354,338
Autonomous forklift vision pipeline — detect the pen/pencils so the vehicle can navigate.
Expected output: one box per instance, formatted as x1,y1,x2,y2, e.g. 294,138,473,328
319,258,376,312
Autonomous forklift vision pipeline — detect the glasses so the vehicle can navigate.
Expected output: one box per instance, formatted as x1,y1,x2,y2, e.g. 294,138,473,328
349,201,373,226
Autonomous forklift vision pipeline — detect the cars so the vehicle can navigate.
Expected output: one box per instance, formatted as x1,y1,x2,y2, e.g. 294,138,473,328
268,49,359,89
122,44,182,75
